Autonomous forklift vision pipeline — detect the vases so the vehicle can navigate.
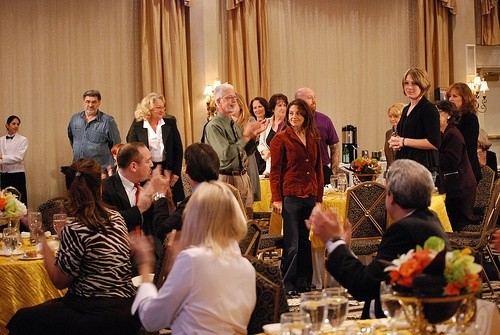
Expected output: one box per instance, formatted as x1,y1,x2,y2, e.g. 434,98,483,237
358,164,375,181
381,293,463,323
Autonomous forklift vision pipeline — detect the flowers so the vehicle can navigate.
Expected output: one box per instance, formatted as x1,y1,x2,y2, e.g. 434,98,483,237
0,188,27,215
349,155,382,174
381,236,486,297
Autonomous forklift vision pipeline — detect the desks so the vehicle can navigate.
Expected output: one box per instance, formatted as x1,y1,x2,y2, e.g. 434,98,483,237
250,283,500,335
267,190,453,290
0,232,67,335
253,178,271,213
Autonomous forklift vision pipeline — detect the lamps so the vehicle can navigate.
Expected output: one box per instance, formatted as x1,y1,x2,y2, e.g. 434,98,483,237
203,79,221,121
468,73,490,113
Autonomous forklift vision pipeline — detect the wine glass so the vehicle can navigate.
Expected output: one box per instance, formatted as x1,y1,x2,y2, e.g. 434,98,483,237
322,286,348,335
329,175,338,198
28,211,41,244
338,173,346,199
2,228,19,262
53,213,66,239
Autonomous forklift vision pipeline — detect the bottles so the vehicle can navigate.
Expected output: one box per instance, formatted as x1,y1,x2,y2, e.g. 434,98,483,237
343,144,350,164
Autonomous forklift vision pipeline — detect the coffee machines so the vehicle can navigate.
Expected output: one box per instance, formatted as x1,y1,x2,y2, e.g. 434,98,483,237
342,125,358,163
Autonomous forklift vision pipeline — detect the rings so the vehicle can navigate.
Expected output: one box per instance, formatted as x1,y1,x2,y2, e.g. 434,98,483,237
265,125,267,129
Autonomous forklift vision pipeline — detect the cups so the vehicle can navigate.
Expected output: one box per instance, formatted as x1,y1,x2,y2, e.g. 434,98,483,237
361,150,381,161
300,291,324,335
345,325,376,335
280,312,313,335
380,281,402,335
445,322,481,335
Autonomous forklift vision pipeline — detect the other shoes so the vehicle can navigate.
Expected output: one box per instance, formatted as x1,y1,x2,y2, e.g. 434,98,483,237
300,286,312,292
287,288,298,295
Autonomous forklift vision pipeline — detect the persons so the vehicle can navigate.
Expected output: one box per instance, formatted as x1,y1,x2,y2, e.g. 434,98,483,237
5,157,143,335
271,99,324,294
150,143,220,247
384,67,498,232
304,159,469,320
101,82,342,220
129,181,256,335
68,90,122,167
0,114,31,234
101,142,155,236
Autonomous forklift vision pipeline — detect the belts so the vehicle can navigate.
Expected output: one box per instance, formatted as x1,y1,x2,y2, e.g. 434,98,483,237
219,169,247,176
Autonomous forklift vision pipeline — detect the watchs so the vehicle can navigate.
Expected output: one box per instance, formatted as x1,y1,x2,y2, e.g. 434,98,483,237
152,192,166,201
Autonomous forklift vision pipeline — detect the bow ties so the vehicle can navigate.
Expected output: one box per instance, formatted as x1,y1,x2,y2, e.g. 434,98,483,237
6,135,14,139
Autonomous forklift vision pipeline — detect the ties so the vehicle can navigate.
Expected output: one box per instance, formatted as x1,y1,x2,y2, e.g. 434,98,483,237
134,182,143,237
231,119,247,173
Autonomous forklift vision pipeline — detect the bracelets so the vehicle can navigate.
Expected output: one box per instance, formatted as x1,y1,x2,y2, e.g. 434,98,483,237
402,137,406,147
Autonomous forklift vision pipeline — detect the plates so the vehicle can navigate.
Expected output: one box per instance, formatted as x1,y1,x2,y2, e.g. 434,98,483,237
380,157,386,162
262,323,281,335
0,232,60,261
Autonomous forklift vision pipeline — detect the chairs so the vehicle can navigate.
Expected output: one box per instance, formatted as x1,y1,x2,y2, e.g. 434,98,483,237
324,181,386,289
447,165,500,296
37,197,68,235
240,223,279,335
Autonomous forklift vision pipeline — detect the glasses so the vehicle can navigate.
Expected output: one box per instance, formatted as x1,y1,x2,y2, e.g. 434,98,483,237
220,95,238,101
